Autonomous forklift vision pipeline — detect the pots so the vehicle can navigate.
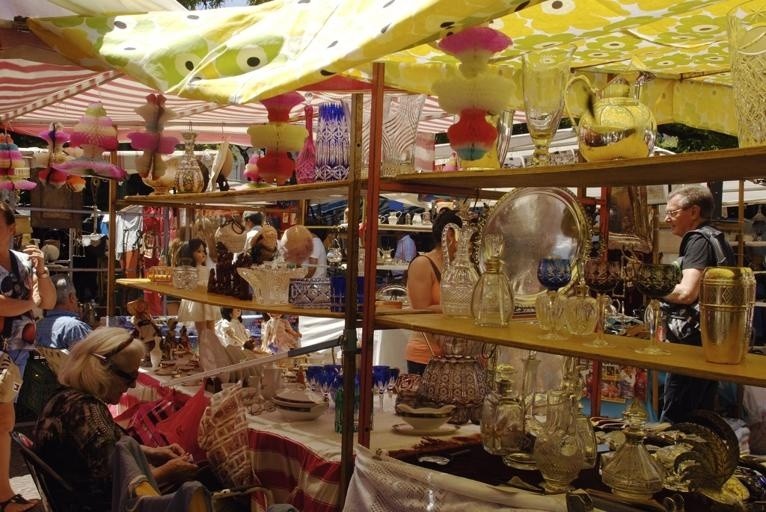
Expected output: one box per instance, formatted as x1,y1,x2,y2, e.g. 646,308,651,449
271,386,330,421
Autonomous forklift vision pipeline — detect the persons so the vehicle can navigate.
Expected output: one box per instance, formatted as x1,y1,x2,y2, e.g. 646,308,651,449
661,185,742,419
307,220,328,278
394,229,417,261
261,312,302,352
404,208,463,374
178,239,217,345
1,201,58,511
215,307,262,353
241,210,266,257
30,327,215,512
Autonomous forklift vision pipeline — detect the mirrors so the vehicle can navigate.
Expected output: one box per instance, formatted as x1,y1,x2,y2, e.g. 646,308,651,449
470,185,592,316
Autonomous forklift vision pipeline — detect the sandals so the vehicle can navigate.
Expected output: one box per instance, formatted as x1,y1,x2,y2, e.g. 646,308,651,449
0,494,40,512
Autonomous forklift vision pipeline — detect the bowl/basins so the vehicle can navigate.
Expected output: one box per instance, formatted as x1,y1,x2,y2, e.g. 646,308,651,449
396,404,458,429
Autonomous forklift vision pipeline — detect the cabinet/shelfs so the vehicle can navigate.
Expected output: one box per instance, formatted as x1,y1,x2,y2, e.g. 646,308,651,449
368,57,765,512
114,95,362,511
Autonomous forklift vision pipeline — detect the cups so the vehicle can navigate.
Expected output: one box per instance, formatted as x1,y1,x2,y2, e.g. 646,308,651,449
378,215,386,224
727,0,766,148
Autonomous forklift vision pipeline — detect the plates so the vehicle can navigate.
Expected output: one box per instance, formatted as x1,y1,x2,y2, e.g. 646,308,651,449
391,423,460,436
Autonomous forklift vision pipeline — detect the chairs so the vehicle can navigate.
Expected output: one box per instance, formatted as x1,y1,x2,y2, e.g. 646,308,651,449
11,430,275,512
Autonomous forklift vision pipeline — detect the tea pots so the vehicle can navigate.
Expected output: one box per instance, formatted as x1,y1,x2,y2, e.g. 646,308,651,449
388,211,402,224
563,71,656,163
440,219,479,318
264,361,288,396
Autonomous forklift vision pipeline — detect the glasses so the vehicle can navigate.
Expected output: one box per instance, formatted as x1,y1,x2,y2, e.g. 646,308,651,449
663,208,687,216
108,363,137,385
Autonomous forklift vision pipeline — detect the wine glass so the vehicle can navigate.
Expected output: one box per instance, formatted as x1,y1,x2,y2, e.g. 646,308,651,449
634,262,677,355
576,257,622,349
521,44,578,168
318,373,334,409
371,365,390,418
385,368,400,411
537,257,572,341
306,365,323,392
323,364,341,387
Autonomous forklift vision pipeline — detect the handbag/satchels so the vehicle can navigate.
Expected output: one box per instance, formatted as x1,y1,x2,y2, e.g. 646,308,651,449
0,248,24,402
665,228,725,343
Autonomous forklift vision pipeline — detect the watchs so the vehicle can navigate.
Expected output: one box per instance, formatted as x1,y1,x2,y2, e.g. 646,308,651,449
38,264,51,277
